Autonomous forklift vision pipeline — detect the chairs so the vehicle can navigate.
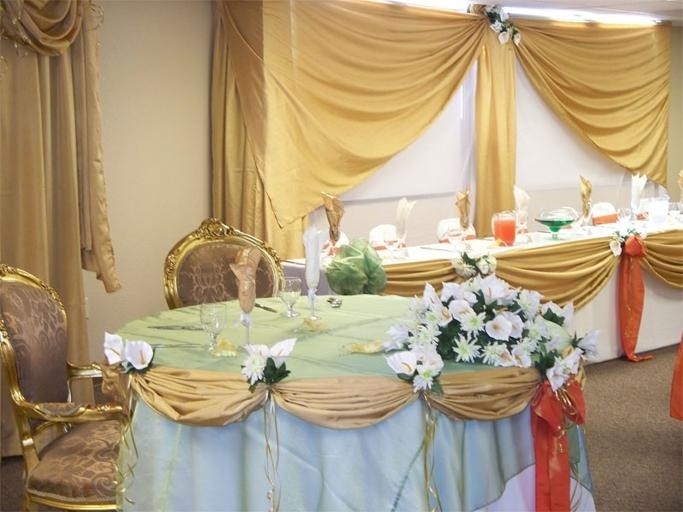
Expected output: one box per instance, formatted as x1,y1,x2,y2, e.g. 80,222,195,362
369,224,406,251
492,212,527,236
164,217,286,309
588,202,618,223
320,231,348,253
437,217,477,242
0,264,131,512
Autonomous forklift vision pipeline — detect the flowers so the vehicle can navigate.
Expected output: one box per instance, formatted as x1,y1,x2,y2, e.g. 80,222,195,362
103,331,154,374
452,253,497,277
609,223,644,257
384,274,596,394
489,4,520,47
242,338,296,392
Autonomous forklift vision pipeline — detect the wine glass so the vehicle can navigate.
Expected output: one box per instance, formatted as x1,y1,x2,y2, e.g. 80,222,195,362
305,257,321,322
395,219,408,257
517,209,529,243
280,277,302,317
238,279,256,352
200,302,228,359
459,211,472,252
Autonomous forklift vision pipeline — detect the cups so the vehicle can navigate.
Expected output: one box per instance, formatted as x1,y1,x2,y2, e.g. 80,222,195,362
491,211,516,247
617,208,631,223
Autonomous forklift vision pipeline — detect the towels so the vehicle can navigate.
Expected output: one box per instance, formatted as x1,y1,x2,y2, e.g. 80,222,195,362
633,172,647,204
513,184,531,220
455,186,471,227
580,176,592,215
229,246,263,312
301,225,326,285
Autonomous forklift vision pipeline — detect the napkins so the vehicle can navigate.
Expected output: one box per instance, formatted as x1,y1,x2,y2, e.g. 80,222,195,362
580,178,592,216
395,199,415,240
456,189,472,229
513,187,530,225
321,192,350,244
